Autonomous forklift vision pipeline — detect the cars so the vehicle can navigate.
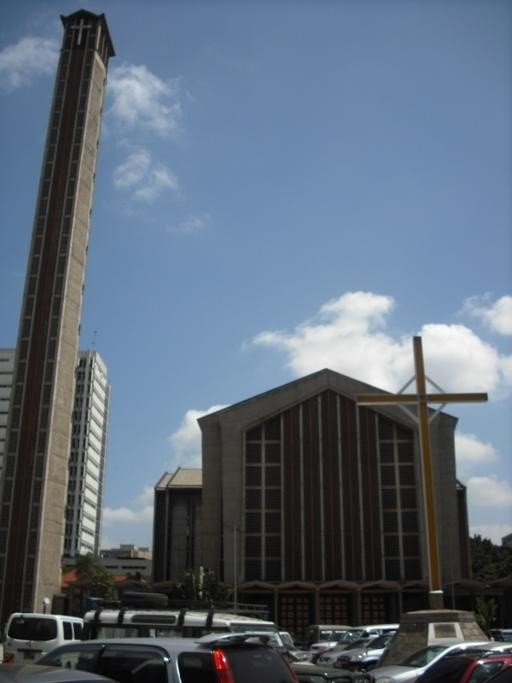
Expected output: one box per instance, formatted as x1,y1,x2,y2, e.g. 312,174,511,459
279,623,512,683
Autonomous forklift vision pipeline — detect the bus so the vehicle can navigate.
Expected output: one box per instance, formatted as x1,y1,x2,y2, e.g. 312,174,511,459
83,607,280,643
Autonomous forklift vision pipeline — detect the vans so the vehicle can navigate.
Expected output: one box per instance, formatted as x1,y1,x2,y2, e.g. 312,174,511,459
32,630,300,683
2,612,83,669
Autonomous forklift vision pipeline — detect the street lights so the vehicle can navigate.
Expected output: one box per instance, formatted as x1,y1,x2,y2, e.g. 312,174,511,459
43,597,50,613
